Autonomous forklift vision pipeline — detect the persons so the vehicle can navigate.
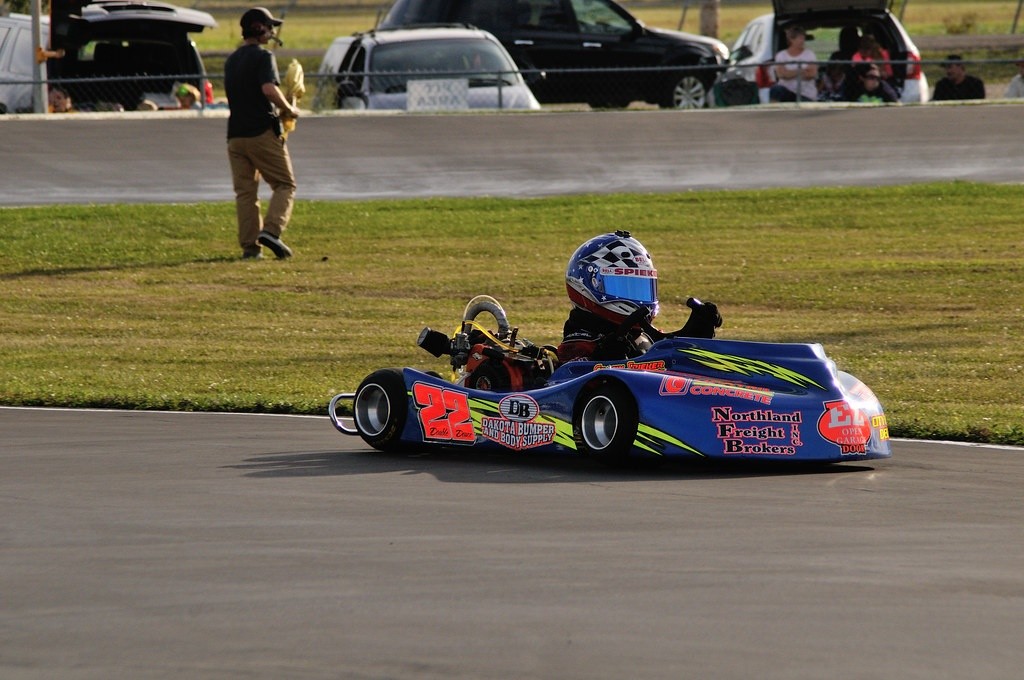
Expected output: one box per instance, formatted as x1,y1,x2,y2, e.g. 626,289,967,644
933,54,985,100
774,28,899,103
175,87,196,109
1006,63,1024,97
557,230,722,362
224,9,300,258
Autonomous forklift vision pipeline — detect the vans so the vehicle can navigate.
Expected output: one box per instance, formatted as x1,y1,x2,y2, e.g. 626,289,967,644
0,0,219,116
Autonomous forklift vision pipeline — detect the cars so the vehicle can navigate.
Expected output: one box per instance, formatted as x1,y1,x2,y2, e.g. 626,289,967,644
707,0,932,109
314,19,543,110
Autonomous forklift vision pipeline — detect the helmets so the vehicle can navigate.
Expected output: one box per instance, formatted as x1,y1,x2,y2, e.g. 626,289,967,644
566,230,660,329
240,6,283,40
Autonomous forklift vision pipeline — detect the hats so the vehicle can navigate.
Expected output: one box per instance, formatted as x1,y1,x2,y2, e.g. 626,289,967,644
943,55,962,65
862,63,880,77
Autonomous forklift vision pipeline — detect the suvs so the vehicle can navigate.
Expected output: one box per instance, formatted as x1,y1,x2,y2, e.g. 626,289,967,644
370,0,730,110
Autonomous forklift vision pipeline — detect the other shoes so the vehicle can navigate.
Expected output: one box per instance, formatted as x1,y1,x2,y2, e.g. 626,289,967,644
257,230,291,258
242,251,262,260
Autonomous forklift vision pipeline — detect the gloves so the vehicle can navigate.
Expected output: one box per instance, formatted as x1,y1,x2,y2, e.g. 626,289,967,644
600,331,632,358
682,302,723,339
